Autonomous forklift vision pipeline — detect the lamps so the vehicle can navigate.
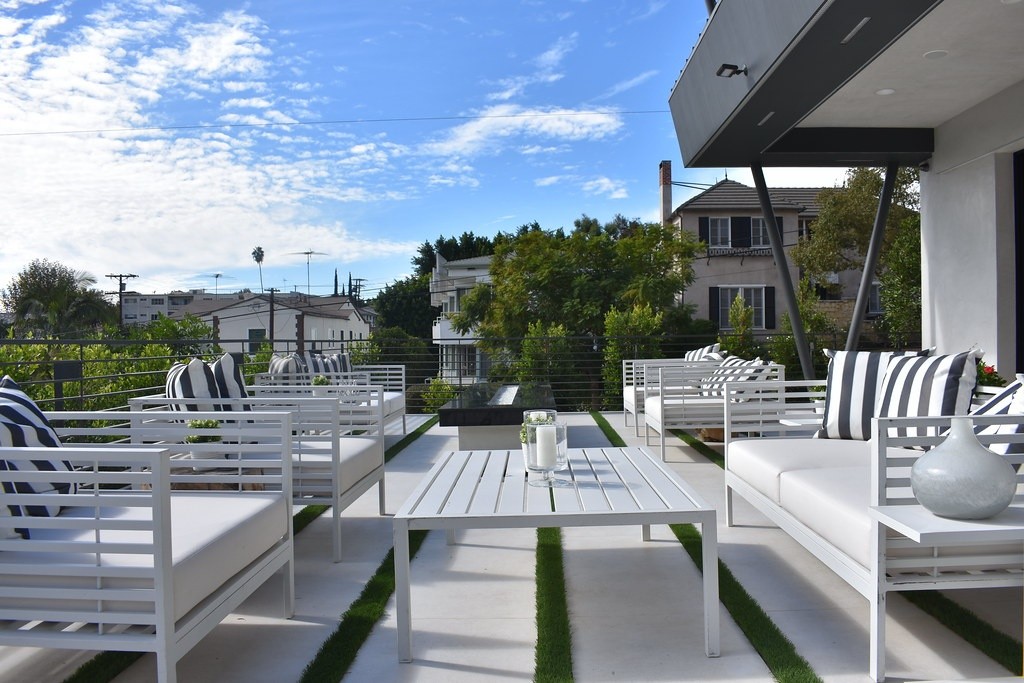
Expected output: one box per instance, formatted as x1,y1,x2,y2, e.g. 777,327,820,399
716,64,748,78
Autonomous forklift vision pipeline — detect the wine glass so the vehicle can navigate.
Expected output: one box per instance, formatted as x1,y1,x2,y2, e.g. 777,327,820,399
526,421,568,487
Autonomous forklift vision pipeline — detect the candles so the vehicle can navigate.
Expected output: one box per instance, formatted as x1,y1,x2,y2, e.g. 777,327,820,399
536,425,557,469
531,411,547,420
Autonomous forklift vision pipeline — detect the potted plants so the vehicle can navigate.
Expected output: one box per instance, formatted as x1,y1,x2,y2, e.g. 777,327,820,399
519,415,554,473
311,375,332,397
186,418,226,472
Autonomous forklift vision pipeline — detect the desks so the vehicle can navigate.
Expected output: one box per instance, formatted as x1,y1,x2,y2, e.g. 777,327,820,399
141,467,265,491
439,382,557,480
392,445,721,665
869,505,1024,544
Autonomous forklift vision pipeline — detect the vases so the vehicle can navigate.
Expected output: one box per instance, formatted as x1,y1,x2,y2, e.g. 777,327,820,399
910,418,1017,520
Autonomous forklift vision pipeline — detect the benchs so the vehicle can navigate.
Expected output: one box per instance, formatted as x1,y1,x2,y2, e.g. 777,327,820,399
644,362,786,463
622,358,723,438
253,364,407,436
125,385,386,564
722,380,1024,683
0,410,296,683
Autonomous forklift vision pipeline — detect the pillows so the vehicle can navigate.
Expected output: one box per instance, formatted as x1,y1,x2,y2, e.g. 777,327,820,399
202,352,259,444
684,342,721,367
700,355,778,404
688,350,728,388
923,380,1024,492
812,346,938,440
269,353,357,407
0,374,79,539
867,348,986,450
165,356,231,459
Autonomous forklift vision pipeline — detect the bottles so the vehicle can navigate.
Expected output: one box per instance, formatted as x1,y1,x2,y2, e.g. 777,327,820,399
910,418,1017,522
